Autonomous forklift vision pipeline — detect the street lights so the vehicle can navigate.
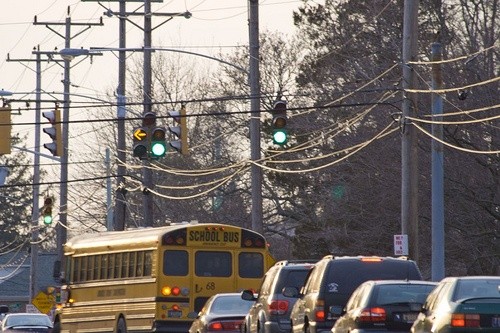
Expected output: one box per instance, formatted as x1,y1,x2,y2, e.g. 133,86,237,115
0,90,153,227
61,48,262,234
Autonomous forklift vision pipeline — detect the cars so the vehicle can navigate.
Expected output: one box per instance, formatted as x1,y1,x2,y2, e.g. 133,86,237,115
411,276,500,333
2,313,54,333
188,293,258,333
329,280,440,333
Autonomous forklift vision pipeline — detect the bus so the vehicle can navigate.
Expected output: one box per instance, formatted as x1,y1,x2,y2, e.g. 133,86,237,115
54,223,277,333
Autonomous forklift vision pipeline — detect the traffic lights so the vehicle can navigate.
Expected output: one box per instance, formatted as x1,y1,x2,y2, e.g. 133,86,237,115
272,100,287,144
167,110,188,155
43,197,53,224
42,110,63,156
133,112,165,160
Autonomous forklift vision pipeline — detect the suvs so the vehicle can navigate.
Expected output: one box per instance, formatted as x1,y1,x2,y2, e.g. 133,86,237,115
241,260,319,333
282,255,422,333
